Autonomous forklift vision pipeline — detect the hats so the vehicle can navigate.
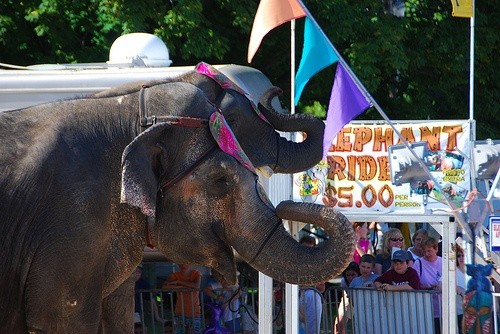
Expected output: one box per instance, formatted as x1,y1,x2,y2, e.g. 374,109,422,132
391,250,409,262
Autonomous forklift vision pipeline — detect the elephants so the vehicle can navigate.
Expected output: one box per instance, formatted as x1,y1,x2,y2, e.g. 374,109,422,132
0,62,357,334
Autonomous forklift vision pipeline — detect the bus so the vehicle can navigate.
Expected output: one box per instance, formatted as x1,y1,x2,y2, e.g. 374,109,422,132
0,32,287,263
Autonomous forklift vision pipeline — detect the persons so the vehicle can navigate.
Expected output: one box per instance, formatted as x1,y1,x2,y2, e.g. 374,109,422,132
350,221,373,263
204,276,243,334
408,229,431,261
373,227,408,277
239,221,361,334
455,245,465,334
369,222,395,252
134,263,177,324
412,237,443,334
162,260,203,334
349,253,382,291
132,310,224,334
373,250,422,291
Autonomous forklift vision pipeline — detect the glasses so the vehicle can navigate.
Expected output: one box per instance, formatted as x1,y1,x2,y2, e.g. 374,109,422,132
457,254,464,257
389,237,403,241
